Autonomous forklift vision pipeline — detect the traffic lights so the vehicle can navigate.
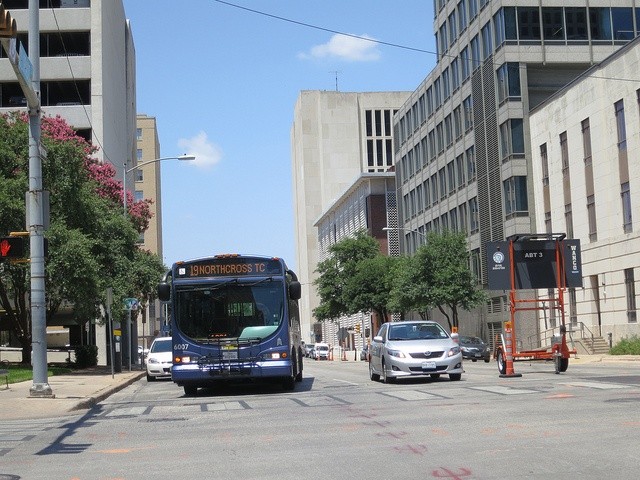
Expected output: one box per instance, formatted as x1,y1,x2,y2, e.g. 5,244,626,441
0,3,17,38
355,323,360,333
0,237,26,259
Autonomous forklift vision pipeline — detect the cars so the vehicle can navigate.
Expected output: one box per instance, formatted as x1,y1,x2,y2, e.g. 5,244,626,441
138,346,149,358
360,345,368,361
368,321,465,383
146,337,172,381
451,336,490,363
309,349,314,359
301,340,305,356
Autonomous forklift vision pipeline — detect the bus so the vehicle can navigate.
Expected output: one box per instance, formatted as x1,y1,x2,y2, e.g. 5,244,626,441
158,254,303,394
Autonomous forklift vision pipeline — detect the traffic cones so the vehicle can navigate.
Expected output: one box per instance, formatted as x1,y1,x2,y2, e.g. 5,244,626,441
450,327,458,343
499,321,522,377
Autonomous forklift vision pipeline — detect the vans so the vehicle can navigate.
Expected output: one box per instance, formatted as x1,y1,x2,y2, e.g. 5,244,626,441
313,343,329,360
302,344,314,358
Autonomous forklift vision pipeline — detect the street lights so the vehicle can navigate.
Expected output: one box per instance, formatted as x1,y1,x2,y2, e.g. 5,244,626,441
123,154,195,217
382,227,426,248
